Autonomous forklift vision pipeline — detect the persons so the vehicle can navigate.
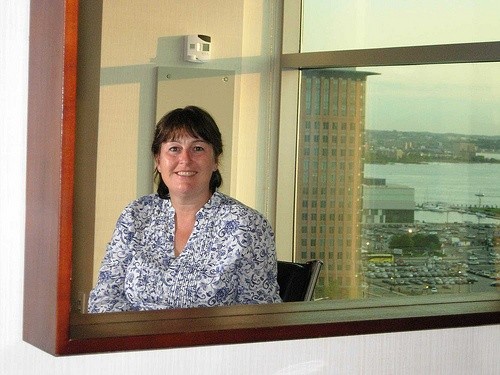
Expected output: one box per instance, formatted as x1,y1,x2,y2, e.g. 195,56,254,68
87,104,284,316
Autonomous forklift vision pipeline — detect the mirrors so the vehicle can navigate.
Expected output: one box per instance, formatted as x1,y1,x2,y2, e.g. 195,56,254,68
70,0,499,326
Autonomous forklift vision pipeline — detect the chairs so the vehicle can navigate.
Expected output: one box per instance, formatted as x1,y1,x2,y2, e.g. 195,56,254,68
277,259,323,302
